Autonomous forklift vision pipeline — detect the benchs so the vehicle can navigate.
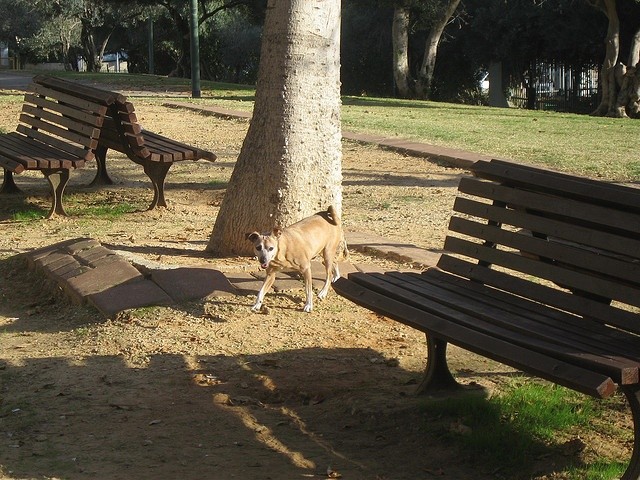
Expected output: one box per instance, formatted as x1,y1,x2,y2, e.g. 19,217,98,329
491,155,640,328
0,73,116,221
330,158,639,479
55,78,218,212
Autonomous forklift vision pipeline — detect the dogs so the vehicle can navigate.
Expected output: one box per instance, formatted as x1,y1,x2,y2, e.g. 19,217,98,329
248,205,343,314
